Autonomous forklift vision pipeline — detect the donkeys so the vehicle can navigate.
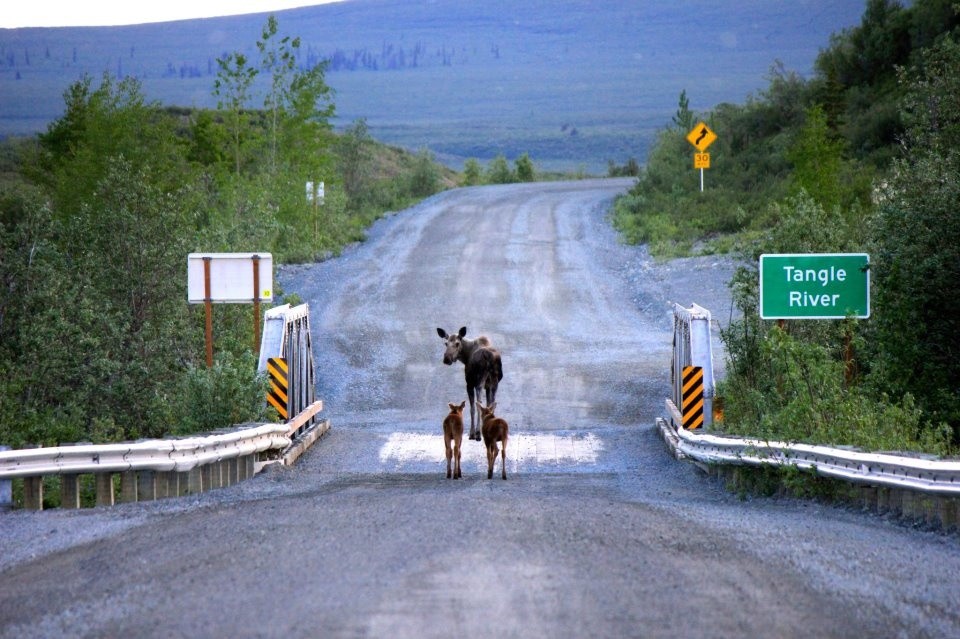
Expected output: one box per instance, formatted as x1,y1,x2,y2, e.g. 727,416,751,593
476,401,508,480
442,400,466,480
436,326,503,440
